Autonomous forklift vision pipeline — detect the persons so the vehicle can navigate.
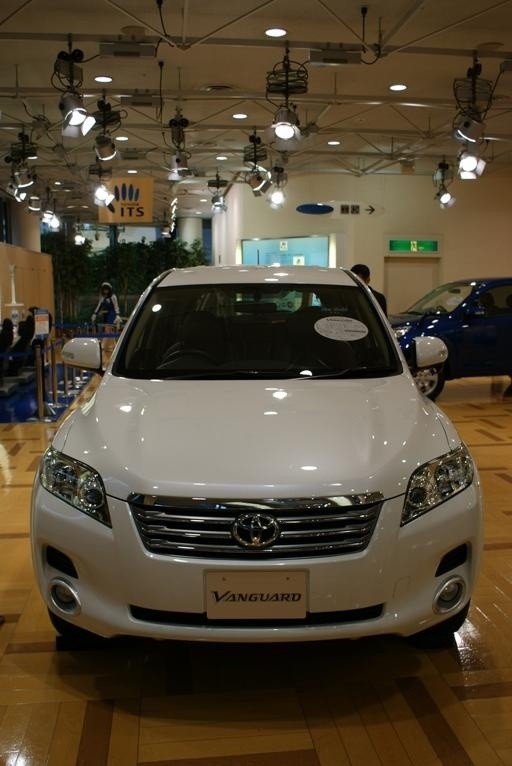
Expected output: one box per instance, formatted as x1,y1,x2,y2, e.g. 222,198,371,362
350,264,387,319
90,281,123,350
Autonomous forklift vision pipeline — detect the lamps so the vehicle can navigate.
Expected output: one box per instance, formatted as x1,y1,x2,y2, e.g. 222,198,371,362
203,106,309,222
429,115,485,211
3,86,195,233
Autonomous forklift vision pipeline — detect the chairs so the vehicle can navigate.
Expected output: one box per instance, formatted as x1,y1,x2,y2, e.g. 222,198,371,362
170,299,332,360
0,314,50,400
480,292,512,315
